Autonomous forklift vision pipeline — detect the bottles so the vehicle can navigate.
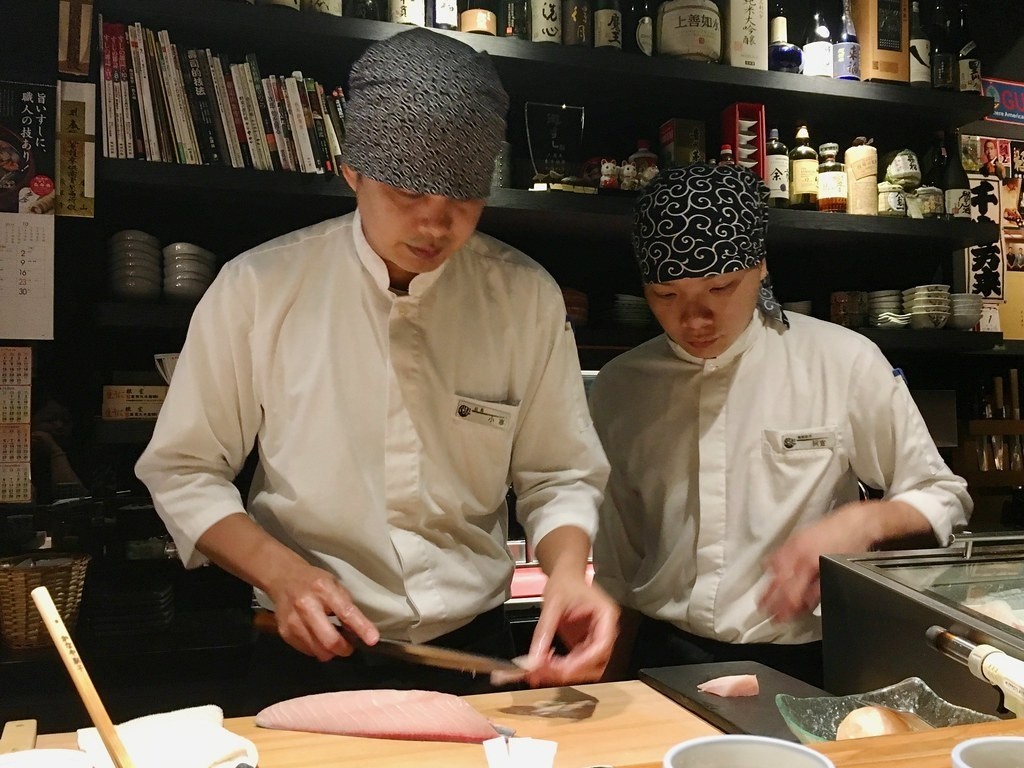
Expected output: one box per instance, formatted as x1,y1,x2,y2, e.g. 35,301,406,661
910,1,931,88
925,135,971,220
768,16,800,75
818,142,846,214
837,11,859,80
803,0,834,78
958,4,982,92
789,125,819,211
764,129,790,209
271,0,654,56
932,1,959,88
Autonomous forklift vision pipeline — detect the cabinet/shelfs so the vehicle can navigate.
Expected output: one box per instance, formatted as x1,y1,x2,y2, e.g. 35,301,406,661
0,0,994,735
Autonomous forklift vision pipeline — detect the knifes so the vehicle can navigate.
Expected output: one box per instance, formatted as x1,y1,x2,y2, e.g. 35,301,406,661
252,612,523,675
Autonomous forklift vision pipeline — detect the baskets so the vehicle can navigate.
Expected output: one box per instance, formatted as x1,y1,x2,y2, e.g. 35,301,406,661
0,552,92,650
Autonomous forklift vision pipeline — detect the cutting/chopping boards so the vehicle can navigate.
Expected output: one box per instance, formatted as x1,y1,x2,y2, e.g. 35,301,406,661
0,679,757,768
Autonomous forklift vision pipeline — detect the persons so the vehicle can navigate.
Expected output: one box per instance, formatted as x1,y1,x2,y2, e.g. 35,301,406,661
133,25,622,715
587,162,974,681
984,140,998,164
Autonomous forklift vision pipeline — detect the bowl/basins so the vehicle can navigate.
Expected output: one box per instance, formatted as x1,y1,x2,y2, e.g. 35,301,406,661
952,291,986,330
163,241,217,302
867,290,900,315
902,284,951,331
110,229,162,302
781,301,813,318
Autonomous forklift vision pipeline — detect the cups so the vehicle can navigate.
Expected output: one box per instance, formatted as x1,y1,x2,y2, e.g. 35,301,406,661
664,734,834,768
952,736,1024,768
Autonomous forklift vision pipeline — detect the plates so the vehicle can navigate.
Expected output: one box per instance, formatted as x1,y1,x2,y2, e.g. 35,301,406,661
869,311,911,329
615,291,651,329
775,674,1004,742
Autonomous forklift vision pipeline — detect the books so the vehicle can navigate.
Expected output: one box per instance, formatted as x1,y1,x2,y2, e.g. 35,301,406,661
98,13,347,177
1,79,96,217
